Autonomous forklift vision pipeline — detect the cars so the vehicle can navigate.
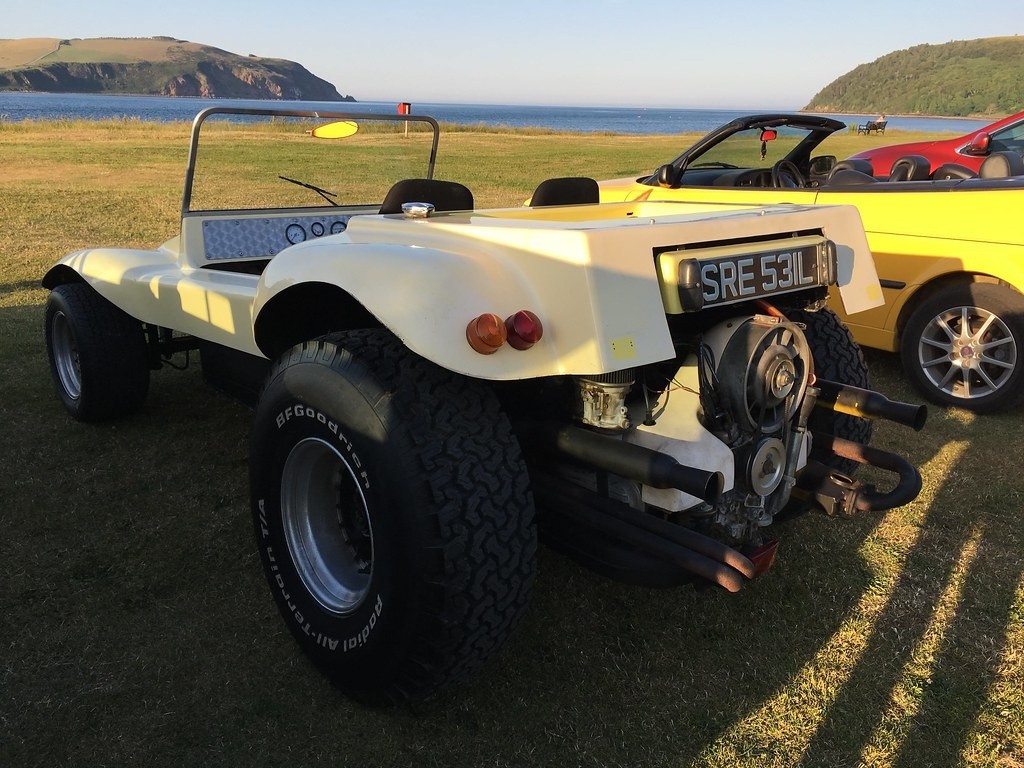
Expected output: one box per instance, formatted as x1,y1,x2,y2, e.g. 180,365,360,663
43,107,928,710
523,113,1023,408
848,112,1024,181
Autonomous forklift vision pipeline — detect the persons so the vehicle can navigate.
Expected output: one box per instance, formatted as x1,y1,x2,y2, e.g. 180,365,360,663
867,114,886,135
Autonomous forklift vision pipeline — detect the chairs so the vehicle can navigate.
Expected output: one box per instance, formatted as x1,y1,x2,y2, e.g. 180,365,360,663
379,179,474,216
529,177,599,208
889,155,930,182
825,159,875,185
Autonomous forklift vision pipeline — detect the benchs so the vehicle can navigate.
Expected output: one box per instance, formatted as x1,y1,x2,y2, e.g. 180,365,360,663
858,120,888,136
